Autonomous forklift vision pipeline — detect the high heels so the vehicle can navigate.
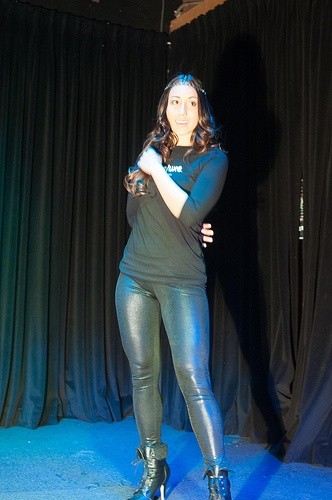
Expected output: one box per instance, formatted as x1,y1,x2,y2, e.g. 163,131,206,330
127,443,171,500
202,468,236,500
